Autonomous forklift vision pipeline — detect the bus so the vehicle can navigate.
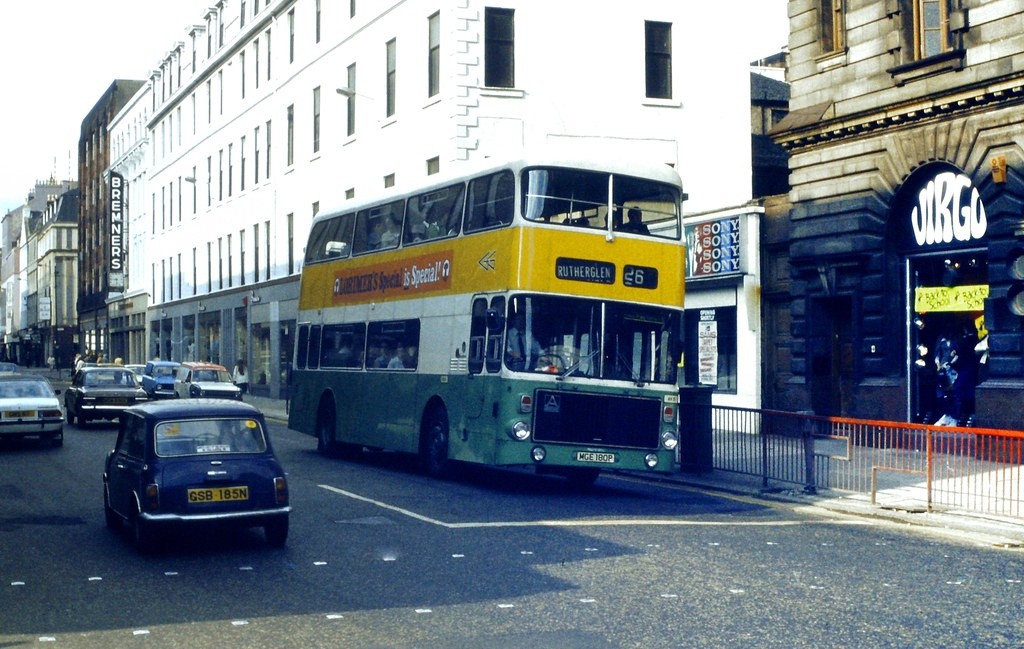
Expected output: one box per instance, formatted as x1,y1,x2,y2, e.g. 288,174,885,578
288,152,689,487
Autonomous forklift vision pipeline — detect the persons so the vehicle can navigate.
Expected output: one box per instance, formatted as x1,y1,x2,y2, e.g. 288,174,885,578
232,359,249,397
624,207,650,234
115,354,124,364
500,313,548,363
427,208,449,239
156,369,164,377
363,214,401,249
563,210,618,228
339,333,353,353
114,372,122,384
367,338,417,368
73,347,109,384
153,354,160,360
47,355,56,371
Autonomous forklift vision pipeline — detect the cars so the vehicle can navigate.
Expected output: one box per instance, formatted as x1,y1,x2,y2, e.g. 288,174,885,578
103,399,292,545
0,371,64,447
1,363,21,380
121,364,146,384
63,367,146,424
174,362,243,402
142,359,181,399
